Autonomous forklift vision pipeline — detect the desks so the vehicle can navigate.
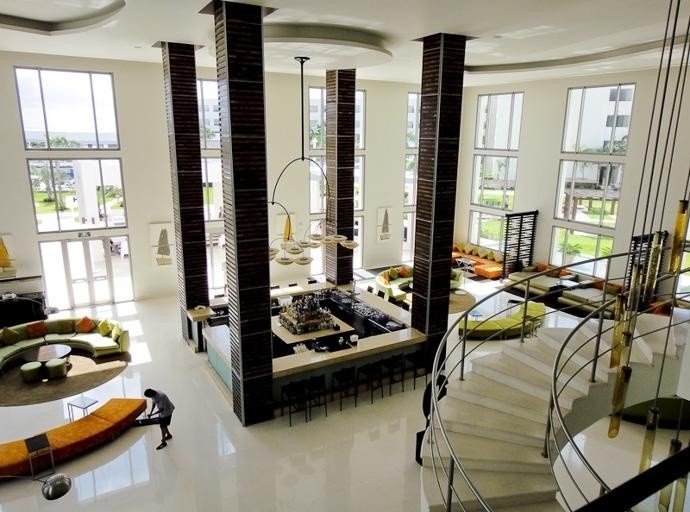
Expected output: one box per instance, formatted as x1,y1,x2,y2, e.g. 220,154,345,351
66,395,99,423
22,344,73,366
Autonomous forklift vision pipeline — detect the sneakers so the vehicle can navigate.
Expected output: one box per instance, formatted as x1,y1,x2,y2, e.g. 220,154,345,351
156,442,167,450
160,434,172,441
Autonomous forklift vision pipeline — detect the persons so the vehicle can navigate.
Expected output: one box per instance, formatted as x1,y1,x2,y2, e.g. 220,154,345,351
144,388,175,450
422,374,448,429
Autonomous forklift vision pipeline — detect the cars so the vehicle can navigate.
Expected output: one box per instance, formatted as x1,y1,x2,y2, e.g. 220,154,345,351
100,218,128,257
207,232,221,248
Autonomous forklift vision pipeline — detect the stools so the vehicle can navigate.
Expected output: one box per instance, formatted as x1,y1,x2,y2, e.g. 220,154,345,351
20,362,42,383
45,359,67,380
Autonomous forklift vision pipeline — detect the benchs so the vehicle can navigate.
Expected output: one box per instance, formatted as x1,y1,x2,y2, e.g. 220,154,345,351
0,399,147,482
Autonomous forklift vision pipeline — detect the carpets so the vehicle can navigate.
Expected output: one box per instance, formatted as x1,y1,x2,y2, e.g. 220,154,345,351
0,346,129,407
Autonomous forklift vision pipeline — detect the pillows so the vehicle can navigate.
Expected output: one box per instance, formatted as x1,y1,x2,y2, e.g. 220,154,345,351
75,315,96,333
0,327,20,345
25,320,48,339
98,317,111,336
111,323,121,341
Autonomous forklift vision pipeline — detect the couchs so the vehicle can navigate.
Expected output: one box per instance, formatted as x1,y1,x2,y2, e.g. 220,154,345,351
368,242,666,342
0,318,130,371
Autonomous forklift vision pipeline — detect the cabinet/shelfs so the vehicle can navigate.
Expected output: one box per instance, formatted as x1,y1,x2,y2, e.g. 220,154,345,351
1,292,49,329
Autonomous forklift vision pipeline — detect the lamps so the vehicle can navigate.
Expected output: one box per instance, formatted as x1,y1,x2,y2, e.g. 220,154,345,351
0,296,61,316
608,1,690,511
0,474,72,501
266,53,360,265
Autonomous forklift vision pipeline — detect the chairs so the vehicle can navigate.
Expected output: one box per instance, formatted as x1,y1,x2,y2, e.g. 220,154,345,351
280,347,427,427
